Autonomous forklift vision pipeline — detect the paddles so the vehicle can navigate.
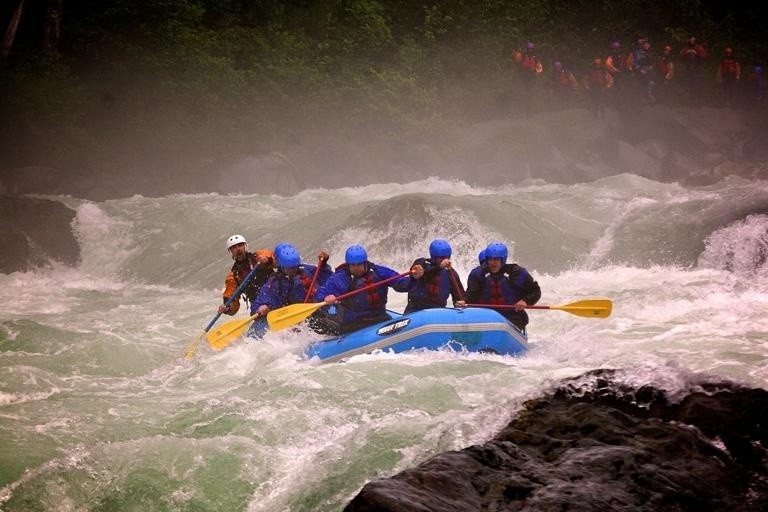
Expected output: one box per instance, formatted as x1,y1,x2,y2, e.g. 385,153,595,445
208,304,272,350
172,259,265,359
267,268,418,330
455,300,612,318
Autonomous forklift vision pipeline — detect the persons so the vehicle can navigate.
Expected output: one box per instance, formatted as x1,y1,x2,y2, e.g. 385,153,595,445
217,235,275,316
456,242,541,332
402,240,466,315
316,246,424,336
496,33,768,120
247,244,334,339
467,248,489,280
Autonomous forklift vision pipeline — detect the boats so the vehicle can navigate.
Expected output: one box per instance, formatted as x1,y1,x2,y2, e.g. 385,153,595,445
246,307,528,362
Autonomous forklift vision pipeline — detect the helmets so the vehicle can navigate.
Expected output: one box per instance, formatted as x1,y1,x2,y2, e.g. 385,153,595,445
479,250,487,265
273,243,300,277
429,240,452,263
486,242,507,264
346,245,367,276
226,235,248,260
